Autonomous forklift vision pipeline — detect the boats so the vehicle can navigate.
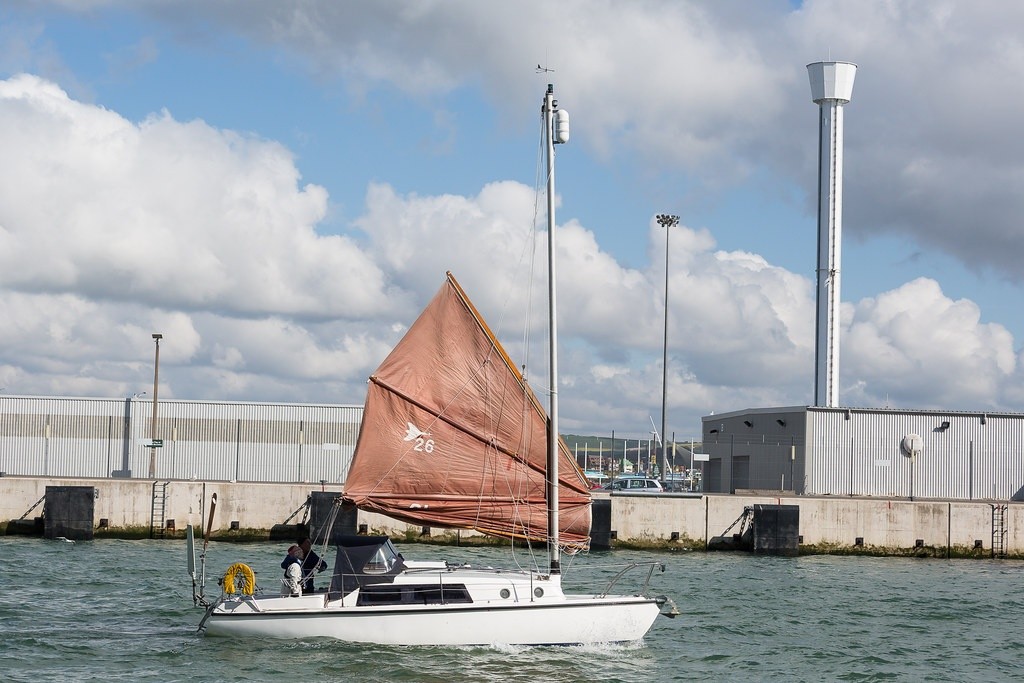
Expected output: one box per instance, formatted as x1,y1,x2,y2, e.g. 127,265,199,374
270,480,389,546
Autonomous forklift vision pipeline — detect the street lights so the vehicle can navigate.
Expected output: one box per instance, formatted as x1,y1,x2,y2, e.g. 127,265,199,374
656,214,680,490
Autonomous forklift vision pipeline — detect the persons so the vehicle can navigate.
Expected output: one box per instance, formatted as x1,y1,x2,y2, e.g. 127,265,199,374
280,536,328,597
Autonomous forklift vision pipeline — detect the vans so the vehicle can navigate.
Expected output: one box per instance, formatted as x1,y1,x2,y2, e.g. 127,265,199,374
592,477,664,492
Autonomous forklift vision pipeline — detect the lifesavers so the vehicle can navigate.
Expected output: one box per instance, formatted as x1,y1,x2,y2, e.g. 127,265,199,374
224,563,255,595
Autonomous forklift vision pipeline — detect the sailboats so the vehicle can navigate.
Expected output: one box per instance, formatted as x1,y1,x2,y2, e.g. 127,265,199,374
185,63,677,648
